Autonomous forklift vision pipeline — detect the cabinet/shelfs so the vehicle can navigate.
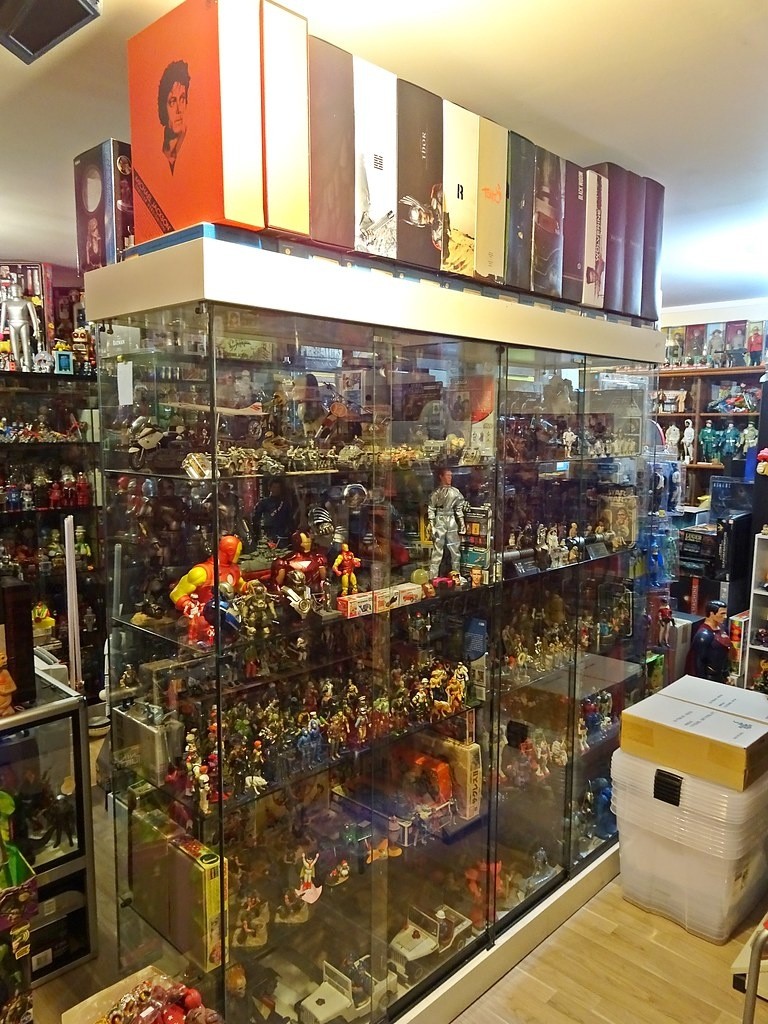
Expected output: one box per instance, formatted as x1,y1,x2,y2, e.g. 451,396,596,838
743,532,768,690
79,237,667,1024
618,365,765,506
0,370,210,705
0,667,100,990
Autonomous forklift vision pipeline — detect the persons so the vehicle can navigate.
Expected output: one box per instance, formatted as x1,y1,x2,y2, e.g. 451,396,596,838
674,326,763,366
613,508,631,543
49,795,75,849
656,594,675,648
120,664,137,708
462,853,498,930
158,60,191,176
691,601,732,684
107,469,482,641
111,373,328,447
398,184,444,251
0,327,91,568
501,400,636,877
338,949,367,1002
0,284,40,370
81,218,102,272
648,387,758,517
585,260,603,288
182,634,469,942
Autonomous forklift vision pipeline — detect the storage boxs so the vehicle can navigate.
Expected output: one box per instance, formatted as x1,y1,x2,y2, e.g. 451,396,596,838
73,0,667,322
55,351,73,374
568,459,768,944
112,329,499,973
